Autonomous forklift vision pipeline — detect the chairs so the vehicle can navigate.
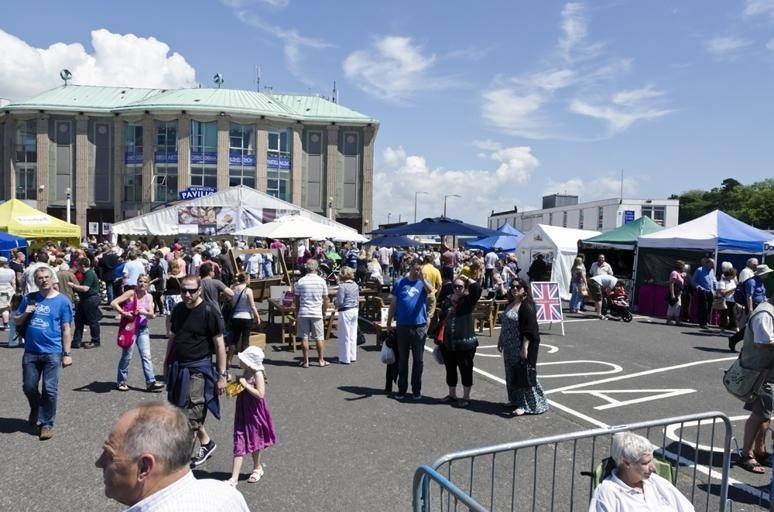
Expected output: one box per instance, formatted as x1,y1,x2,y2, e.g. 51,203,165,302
268,285,295,328
473,298,499,339
593,456,674,490
358,276,389,320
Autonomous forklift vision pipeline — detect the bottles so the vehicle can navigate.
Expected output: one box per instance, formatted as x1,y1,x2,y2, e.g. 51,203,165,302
281,290,286,305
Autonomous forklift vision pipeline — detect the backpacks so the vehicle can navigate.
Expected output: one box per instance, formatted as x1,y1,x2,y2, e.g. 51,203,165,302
734,277,757,305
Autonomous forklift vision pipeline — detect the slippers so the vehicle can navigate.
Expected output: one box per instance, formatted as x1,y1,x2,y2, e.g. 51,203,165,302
457,398,469,407
299,361,308,367
440,395,452,401
320,361,331,367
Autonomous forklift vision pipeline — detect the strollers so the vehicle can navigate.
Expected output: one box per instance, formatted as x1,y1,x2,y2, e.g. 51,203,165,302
602,283,634,323
320,252,350,284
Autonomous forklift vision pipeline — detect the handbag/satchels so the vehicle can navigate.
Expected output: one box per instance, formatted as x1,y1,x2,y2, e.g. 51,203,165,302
84,307,103,325
579,288,589,297
15,293,34,336
222,302,232,324
117,304,140,349
665,293,679,306
434,321,446,345
723,358,770,404
519,358,536,387
712,297,728,309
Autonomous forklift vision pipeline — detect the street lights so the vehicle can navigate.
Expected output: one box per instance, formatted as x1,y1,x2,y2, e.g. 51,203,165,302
327,195,334,218
387,212,391,224
65,187,73,222
415,190,430,224
444,192,464,218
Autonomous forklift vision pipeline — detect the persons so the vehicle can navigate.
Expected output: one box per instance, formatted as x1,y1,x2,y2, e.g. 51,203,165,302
588,432,696,512
1,235,548,488
587,274,625,320
665,257,774,352
588,254,614,316
569,256,587,315
735,270,774,474
95,399,251,512
568,253,587,311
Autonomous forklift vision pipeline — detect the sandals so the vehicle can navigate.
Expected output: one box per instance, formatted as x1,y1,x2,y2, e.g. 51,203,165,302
753,452,773,467
737,454,765,474
225,479,238,488
597,315,608,320
247,467,264,482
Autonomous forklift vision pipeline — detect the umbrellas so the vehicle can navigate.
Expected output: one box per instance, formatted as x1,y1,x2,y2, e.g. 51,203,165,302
364,215,518,266
228,213,370,315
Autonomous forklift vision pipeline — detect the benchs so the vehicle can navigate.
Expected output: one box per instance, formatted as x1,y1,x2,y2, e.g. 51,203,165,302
245,280,285,302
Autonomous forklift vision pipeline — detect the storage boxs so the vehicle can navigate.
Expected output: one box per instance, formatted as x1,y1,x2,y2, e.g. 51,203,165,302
235,332,267,354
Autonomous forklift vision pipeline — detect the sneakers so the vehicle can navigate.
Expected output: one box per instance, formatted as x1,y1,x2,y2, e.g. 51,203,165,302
84,342,100,349
666,321,673,325
192,439,217,465
395,393,405,399
702,325,709,329
158,314,163,317
117,384,129,391
412,392,421,399
40,428,52,439
146,384,165,392
728,336,735,350
28,414,37,425
71,341,81,348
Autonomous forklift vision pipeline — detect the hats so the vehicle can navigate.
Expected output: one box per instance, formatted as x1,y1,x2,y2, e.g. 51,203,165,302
237,345,265,370
754,264,774,276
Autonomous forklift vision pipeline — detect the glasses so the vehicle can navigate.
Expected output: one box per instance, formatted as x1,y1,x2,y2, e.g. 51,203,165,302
511,284,522,290
181,288,198,293
453,284,463,289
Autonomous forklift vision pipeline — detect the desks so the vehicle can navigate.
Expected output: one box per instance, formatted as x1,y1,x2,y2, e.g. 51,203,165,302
476,300,510,328
267,297,339,353
